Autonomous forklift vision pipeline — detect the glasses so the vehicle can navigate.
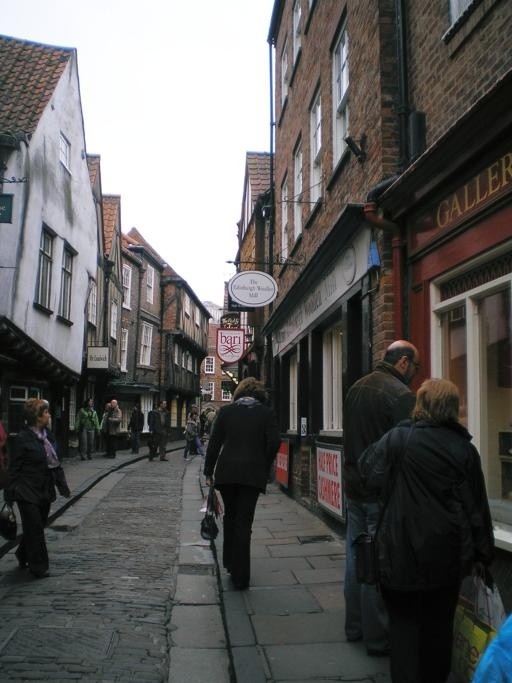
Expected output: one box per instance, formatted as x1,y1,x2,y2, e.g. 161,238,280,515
412,360,420,369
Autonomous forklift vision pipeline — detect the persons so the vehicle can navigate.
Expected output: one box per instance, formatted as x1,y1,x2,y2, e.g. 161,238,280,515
127,403,144,454
75,397,101,461
3,399,72,578
104,399,122,458
201,408,216,434
146,404,164,461
101,402,113,456
202,377,281,593
183,406,204,461
158,399,171,461
342,340,422,658
355,377,495,683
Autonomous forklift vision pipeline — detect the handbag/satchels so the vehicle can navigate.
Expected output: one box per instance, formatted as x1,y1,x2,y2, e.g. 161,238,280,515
1,501,16,541
201,479,218,540
451,564,495,682
